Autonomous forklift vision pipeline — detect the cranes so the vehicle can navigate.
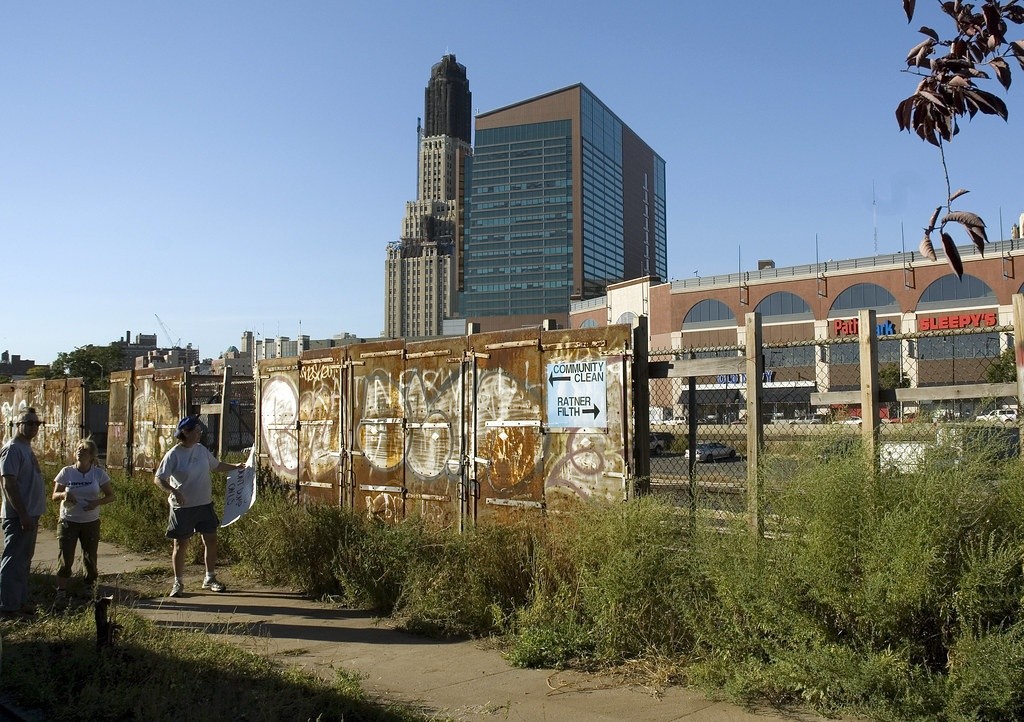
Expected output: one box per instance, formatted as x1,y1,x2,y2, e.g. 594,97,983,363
154,313,181,350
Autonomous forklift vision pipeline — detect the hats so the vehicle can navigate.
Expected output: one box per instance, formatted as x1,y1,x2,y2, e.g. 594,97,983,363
174,416,196,439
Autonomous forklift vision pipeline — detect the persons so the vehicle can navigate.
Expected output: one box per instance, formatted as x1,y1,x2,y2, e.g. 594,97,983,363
154,416,246,596
52,438,114,597
0,406,45,612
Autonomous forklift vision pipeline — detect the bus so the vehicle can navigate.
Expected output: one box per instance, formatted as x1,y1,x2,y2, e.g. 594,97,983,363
827,403,896,420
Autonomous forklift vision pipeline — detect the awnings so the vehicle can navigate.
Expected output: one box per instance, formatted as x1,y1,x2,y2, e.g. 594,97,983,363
677,385,896,404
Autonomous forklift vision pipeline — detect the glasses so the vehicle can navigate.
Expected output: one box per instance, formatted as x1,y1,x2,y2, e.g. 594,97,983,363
20,420,40,427
194,429,203,434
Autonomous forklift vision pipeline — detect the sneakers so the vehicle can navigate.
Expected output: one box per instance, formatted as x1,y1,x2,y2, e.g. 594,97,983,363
170,583,184,597
202,577,226,591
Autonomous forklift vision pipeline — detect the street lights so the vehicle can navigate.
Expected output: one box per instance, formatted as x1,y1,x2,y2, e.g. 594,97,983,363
90,361,103,405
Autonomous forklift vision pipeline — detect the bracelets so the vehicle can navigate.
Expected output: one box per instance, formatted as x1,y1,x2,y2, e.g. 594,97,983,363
60,492,61,499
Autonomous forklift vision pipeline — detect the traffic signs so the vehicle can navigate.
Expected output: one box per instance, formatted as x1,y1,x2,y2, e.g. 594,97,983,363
546,361,608,428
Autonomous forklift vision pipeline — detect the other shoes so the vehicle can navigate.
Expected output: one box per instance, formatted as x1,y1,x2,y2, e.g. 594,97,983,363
0,607,35,619
56,591,66,608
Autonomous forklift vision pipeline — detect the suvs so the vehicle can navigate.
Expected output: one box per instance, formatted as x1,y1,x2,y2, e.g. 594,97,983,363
975,409,1019,422
633,433,665,455
789,414,827,425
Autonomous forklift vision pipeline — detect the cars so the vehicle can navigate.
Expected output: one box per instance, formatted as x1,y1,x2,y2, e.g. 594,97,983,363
662,416,686,425
685,442,736,463
890,414,918,423
701,418,716,425
731,418,747,424
831,416,862,425
